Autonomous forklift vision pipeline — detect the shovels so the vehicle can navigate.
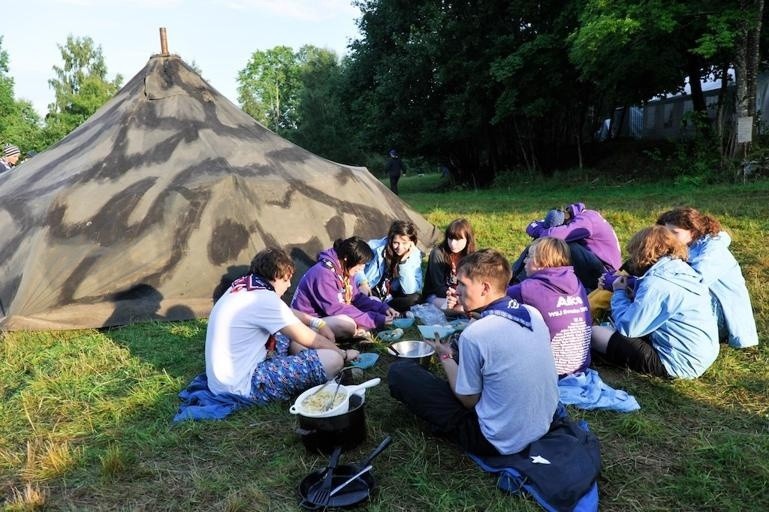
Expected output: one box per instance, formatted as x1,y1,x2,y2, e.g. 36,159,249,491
311,447,341,505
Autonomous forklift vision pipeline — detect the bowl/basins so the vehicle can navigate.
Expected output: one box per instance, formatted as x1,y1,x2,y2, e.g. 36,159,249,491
451,318,469,330
290,385,368,454
418,324,454,341
393,318,415,328
378,330,403,342
350,353,379,369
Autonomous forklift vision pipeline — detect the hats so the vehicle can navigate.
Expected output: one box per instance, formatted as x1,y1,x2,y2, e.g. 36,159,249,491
544,210,564,229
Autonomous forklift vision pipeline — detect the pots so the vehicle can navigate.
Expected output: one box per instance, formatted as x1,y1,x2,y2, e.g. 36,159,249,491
289,376,381,417
297,436,394,510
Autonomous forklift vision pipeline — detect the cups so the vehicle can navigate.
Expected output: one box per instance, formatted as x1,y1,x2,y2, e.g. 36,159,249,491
604,271,635,295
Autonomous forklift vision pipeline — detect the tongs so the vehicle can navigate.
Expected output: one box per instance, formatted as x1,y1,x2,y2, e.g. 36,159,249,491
301,371,346,410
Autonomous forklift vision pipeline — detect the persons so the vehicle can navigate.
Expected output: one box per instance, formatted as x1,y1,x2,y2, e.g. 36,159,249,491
590,224,721,380
0,144,20,174
507,202,622,288
290,235,403,334
427,217,476,297
384,148,407,194
656,204,761,352
385,247,559,460
21,150,37,165
506,235,592,380
363,220,424,312
202,246,365,410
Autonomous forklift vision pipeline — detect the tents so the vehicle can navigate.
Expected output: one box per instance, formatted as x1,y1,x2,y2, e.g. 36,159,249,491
0,53,446,335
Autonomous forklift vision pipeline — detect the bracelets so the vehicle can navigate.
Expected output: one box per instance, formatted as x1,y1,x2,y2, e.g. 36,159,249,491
439,354,453,361
309,317,326,330
342,349,347,360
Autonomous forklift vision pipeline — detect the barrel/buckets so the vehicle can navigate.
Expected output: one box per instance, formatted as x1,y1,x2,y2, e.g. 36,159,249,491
289,383,366,453
